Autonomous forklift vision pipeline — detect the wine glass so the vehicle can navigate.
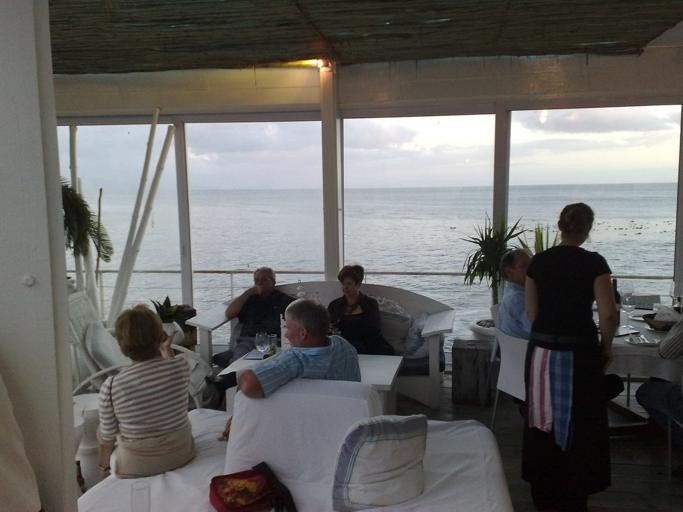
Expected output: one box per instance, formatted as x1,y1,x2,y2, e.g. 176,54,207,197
670,282,683,312
620,281,637,330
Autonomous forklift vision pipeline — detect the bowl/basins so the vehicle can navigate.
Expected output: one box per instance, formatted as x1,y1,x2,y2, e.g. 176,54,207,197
641,312,676,329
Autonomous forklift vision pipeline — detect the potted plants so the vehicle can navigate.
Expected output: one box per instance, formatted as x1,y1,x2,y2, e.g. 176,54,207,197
151,296,184,345
458,212,531,339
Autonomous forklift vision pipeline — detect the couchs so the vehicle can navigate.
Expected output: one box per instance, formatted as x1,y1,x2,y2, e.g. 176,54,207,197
184,282,455,411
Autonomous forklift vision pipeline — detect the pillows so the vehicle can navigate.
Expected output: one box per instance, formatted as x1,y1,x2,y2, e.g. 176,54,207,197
85,319,126,377
374,296,411,338
406,310,428,351
332,412,428,512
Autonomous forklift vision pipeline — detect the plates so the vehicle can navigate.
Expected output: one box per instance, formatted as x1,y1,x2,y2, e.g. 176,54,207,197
624,335,662,346
627,314,646,321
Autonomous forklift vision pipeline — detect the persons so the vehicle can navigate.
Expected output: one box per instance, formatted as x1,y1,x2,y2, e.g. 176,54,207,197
497,250,530,340
525,203,616,510
636,317,683,475
235,299,360,399
223,267,295,366
327,265,393,357
97,304,196,478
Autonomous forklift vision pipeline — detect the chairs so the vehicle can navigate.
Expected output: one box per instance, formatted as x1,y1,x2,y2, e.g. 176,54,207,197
627,296,660,407
491,330,531,427
484,304,503,391
67,290,213,408
667,416,683,483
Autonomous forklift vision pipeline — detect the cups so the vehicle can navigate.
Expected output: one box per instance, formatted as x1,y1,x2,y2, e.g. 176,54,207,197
254,331,277,358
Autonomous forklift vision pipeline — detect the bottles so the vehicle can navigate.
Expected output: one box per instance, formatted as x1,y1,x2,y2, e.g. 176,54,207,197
611,280,621,327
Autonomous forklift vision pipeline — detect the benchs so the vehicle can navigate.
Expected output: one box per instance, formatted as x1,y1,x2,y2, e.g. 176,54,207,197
73,407,516,512
